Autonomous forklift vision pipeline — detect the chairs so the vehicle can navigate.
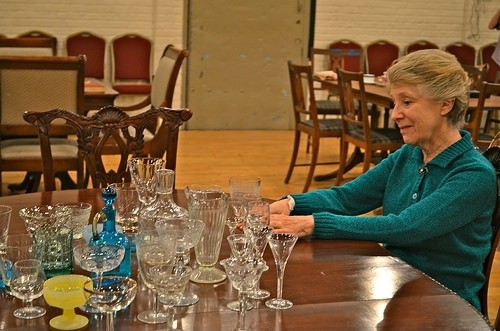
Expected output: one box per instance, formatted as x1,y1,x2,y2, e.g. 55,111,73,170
0,30,193,193
285,40,500,193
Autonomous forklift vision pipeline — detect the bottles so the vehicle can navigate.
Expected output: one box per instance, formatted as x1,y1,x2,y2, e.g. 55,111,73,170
89,187,131,286
139,169,190,241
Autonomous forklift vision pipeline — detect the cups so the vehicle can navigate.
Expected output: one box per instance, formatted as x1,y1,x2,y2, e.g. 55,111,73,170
33,226,73,277
129,157,167,206
229,175,261,197
56,202,92,239
184,184,222,200
363,74,375,84
0,234,46,295
0,204,12,254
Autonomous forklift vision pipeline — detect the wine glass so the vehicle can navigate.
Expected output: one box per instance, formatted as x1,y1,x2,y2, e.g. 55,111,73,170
134,230,188,331
218,257,266,331
10,259,47,319
155,218,205,305
226,234,255,311
43,274,93,330
73,243,125,313
264,233,299,310
83,276,137,331
187,191,228,283
226,194,274,299
108,183,144,230
19,204,62,253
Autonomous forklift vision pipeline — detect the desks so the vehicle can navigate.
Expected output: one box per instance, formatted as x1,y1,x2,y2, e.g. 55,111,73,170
0,188,500,331
84,78,119,110
312,71,500,183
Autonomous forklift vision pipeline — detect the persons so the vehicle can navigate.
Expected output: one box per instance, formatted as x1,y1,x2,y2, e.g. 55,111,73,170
237,48,498,311
